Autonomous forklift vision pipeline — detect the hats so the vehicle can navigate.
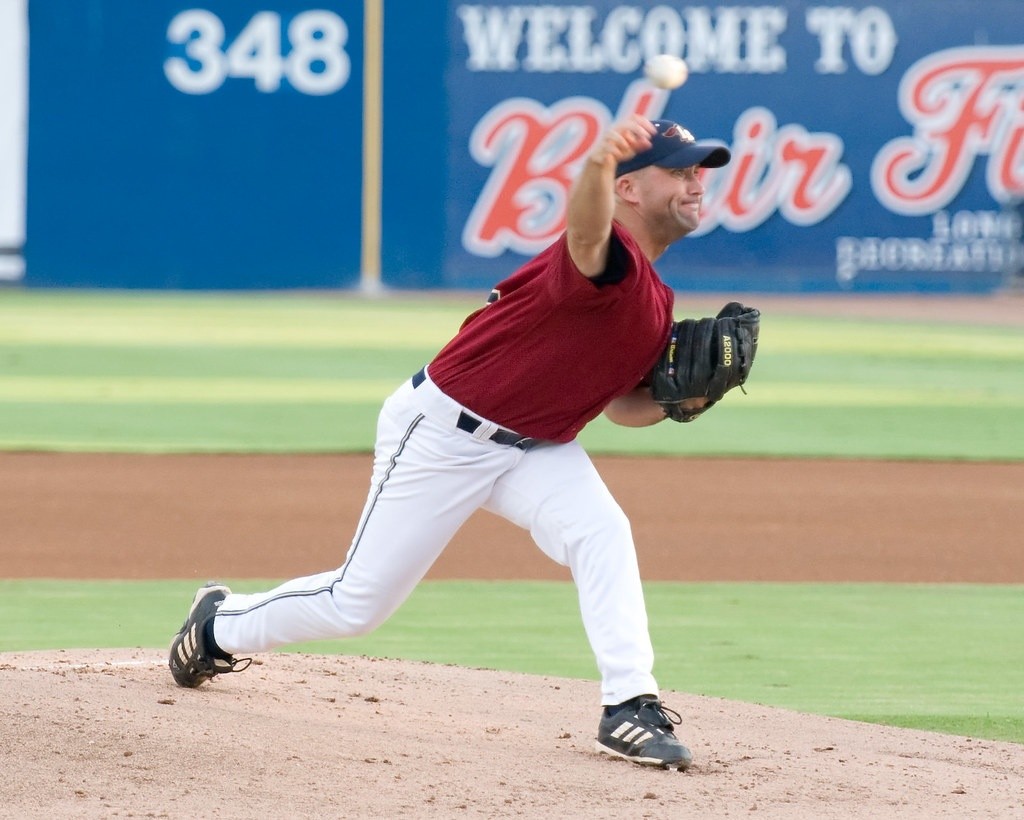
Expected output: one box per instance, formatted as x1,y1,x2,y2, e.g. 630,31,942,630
614,119,731,179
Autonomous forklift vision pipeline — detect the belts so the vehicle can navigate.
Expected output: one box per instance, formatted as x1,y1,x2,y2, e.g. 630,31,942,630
411,365,542,451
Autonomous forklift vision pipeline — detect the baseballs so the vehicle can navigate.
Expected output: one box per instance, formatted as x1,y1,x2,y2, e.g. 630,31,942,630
644,53,690,90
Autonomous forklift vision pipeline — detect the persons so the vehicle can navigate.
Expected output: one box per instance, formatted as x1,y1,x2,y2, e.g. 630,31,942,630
164,113,761,769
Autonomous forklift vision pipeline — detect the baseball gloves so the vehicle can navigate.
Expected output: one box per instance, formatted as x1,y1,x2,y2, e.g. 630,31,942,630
651,302,762,424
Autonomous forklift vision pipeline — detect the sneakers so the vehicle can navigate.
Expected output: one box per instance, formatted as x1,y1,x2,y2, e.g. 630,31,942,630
170,584,252,688
595,697,693,768
